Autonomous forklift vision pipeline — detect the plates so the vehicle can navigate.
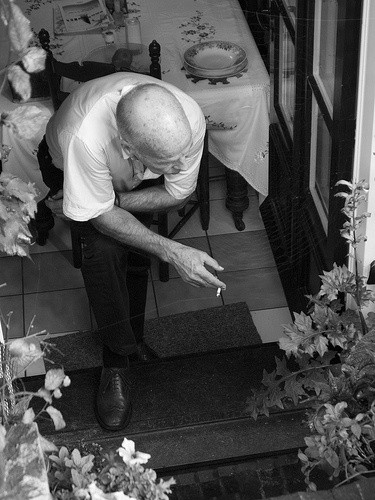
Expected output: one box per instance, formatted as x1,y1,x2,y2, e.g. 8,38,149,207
184,41,248,79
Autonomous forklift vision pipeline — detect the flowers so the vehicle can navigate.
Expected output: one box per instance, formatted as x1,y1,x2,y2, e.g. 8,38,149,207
45,437,177,500
247,179,374,490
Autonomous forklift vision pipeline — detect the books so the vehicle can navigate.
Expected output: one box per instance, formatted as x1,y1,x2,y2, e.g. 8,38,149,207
52,0,114,36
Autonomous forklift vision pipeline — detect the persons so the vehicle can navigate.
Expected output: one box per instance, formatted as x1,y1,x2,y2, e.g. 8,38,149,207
38,71,227,431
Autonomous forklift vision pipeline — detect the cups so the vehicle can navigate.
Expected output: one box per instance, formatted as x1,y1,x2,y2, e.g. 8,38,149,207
103,30,114,45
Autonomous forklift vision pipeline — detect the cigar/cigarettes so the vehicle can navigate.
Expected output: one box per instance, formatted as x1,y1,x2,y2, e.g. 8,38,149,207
215,286,223,297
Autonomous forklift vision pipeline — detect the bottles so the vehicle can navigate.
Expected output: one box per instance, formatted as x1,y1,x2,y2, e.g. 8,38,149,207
124,15,142,55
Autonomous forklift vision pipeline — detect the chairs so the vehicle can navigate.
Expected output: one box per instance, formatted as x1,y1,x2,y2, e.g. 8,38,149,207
39,26,211,284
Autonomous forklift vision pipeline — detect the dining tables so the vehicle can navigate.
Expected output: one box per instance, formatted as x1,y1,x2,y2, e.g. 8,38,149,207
9,0,271,246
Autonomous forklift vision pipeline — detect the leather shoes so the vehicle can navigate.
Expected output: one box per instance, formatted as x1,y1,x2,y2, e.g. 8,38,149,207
129,340,161,373
95,356,133,432
